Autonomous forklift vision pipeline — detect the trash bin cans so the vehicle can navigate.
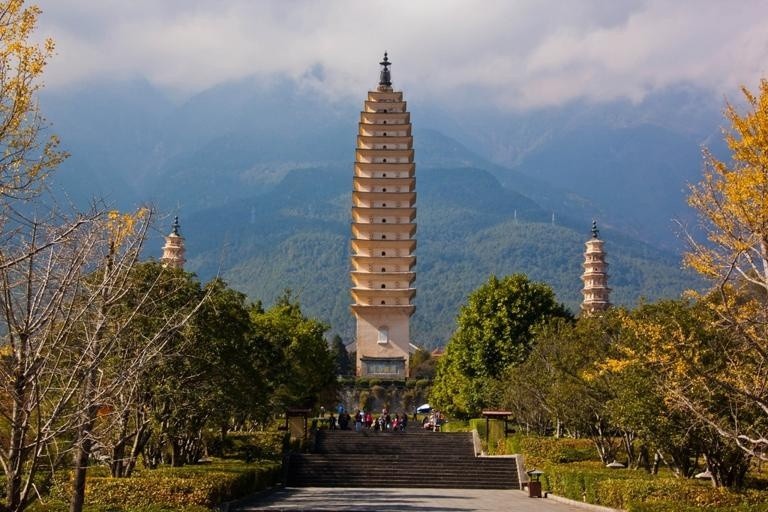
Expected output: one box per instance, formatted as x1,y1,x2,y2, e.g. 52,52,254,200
527,469,544,497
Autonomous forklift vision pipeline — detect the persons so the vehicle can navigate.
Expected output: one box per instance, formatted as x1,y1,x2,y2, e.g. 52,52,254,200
310,406,440,432
